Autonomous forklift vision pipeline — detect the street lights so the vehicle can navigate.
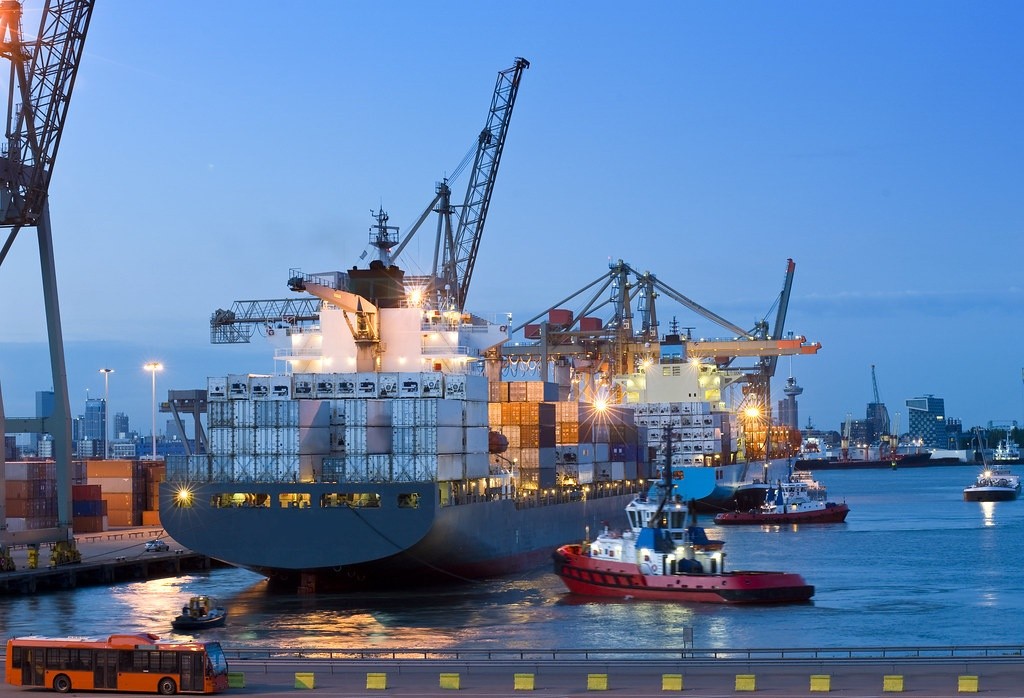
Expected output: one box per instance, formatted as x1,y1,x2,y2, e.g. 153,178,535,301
97,368,114,460
144,362,163,461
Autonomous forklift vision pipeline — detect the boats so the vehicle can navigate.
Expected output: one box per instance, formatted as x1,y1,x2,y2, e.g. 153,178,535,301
172,596,228,631
993,430,1021,465
790,471,828,502
794,416,932,470
714,479,850,525
963,427,1021,502
158,166,804,603
553,424,816,609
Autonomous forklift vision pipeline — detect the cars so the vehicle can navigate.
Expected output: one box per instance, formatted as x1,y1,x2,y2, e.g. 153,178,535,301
145,539,169,552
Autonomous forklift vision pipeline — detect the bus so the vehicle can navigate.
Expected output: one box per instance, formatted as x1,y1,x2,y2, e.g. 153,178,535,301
5,632,229,695
